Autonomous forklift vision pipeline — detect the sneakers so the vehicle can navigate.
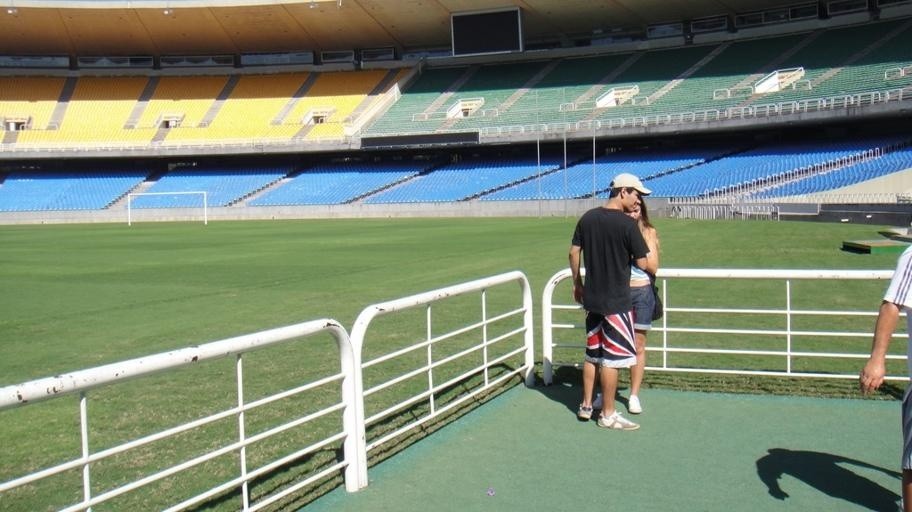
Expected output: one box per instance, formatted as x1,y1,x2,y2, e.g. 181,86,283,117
628,395,643,414
593,393,602,409
597,411,640,431
577,403,594,419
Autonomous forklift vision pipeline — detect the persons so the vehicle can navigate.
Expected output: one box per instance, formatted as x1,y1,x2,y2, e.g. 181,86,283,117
858,241,912,510
591,194,659,416
567,172,649,431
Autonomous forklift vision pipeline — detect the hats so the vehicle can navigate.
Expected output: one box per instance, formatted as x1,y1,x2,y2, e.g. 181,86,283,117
611,173,653,196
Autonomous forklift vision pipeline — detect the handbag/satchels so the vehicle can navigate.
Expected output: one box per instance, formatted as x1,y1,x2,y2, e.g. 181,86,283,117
652,288,663,320
